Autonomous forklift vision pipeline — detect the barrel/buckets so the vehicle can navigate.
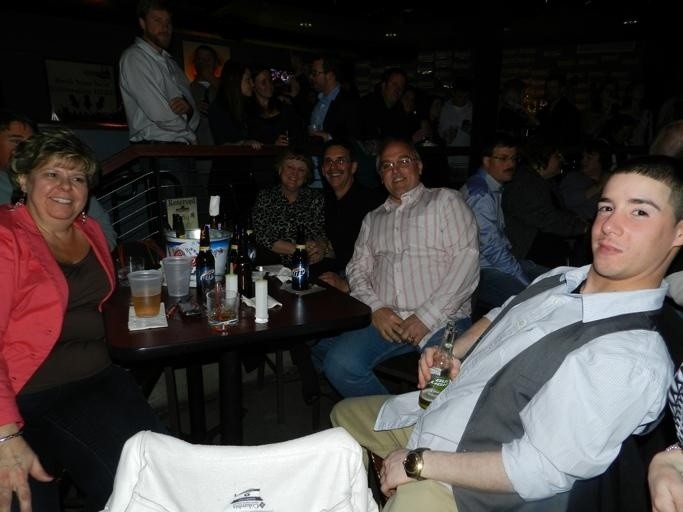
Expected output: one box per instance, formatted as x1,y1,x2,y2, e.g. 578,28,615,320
165,228,234,290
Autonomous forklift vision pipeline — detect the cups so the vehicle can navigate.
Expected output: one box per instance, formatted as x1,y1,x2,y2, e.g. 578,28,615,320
123,256,145,275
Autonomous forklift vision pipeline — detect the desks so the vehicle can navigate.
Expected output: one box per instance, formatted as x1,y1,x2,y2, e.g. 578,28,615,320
104,247,374,442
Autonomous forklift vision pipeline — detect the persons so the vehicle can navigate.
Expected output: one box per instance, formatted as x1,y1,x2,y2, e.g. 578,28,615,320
2,1,683,511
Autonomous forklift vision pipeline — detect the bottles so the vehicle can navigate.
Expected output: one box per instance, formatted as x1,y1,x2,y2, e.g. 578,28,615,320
291,230,309,290
416,320,458,414
194,225,215,308
173,215,187,239
252,272,269,319
226,219,256,300
207,193,220,230
224,263,237,299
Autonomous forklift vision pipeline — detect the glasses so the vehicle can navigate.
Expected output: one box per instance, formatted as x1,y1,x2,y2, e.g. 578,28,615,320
492,155,519,164
322,157,352,166
310,70,326,78
380,159,415,169
